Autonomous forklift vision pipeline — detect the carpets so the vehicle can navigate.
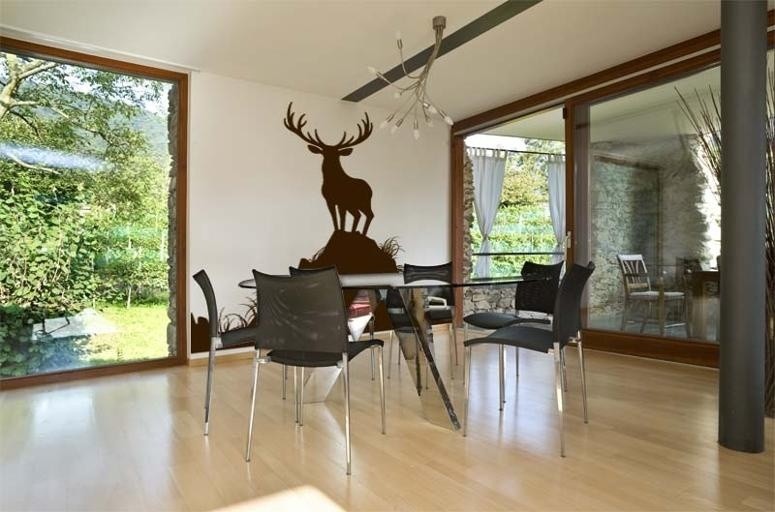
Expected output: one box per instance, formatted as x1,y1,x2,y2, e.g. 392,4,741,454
208,484,345,512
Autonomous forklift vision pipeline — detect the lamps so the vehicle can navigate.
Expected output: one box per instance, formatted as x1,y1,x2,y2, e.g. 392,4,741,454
366,15,453,141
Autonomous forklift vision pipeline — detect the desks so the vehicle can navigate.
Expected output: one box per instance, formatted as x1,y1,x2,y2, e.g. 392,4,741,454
238,273,555,431
690,271,720,345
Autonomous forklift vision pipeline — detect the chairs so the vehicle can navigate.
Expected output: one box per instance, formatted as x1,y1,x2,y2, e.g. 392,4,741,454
245,270,386,474
405,262,459,389
462,261,564,410
616,253,687,339
675,257,703,324
462,262,595,458
192,270,287,436
